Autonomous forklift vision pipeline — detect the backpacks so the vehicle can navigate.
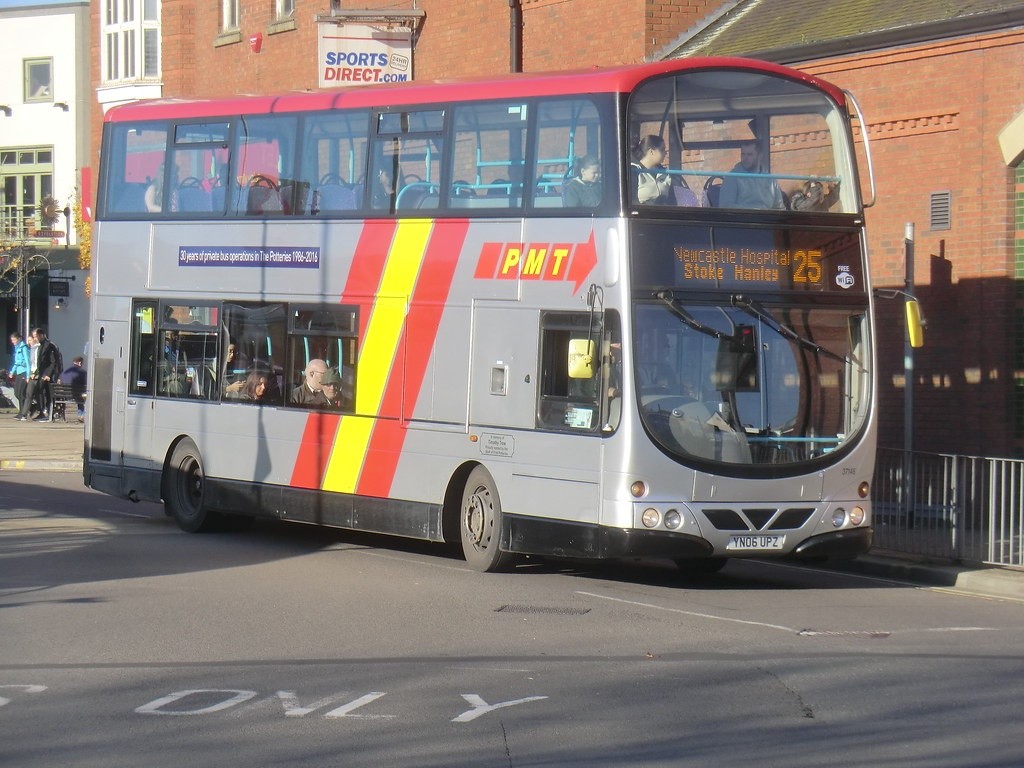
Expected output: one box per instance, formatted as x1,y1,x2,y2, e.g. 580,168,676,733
46,342,64,383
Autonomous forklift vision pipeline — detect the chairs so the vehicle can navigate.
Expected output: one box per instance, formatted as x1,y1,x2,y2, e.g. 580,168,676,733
145,172,724,215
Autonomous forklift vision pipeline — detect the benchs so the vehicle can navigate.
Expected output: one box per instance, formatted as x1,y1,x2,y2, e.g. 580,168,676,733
47,380,87,422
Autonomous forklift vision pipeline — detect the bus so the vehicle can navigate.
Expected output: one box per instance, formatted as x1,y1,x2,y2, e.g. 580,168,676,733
83,56,925,576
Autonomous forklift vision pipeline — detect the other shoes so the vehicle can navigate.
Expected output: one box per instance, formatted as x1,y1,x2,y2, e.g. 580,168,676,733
32,414,45,421
39,417,49,422
14,414,26,421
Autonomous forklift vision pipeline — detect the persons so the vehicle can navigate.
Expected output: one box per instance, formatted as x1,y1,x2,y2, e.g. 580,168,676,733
628,135,685,206
7,327,67,423
371,161,407,212
145,163,181,214
152,316,355,410
787,175,840,212
718,142,786,209
58,356,88,423
563,154,603,207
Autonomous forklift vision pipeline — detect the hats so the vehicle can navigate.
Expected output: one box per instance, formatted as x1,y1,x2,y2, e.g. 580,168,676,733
319,369,340,385
